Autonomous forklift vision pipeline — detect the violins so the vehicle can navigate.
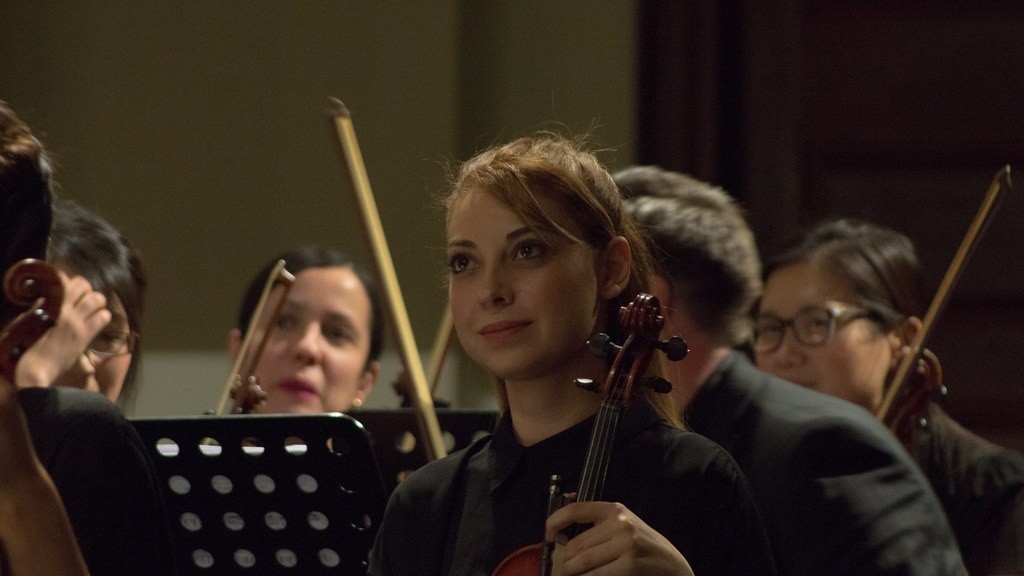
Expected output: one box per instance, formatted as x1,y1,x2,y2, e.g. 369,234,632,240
0,257,66,386
883,348,944,452
489,291,689,576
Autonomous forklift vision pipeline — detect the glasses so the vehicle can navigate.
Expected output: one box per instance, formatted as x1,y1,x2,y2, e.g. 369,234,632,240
751,302,877,354
89,328,135,358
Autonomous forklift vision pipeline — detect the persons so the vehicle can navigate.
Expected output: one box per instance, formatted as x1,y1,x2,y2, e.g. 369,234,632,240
367,135,778,576
228,247,383,447
606,166,968,576
749,218,1024,576
0,103,141,576
